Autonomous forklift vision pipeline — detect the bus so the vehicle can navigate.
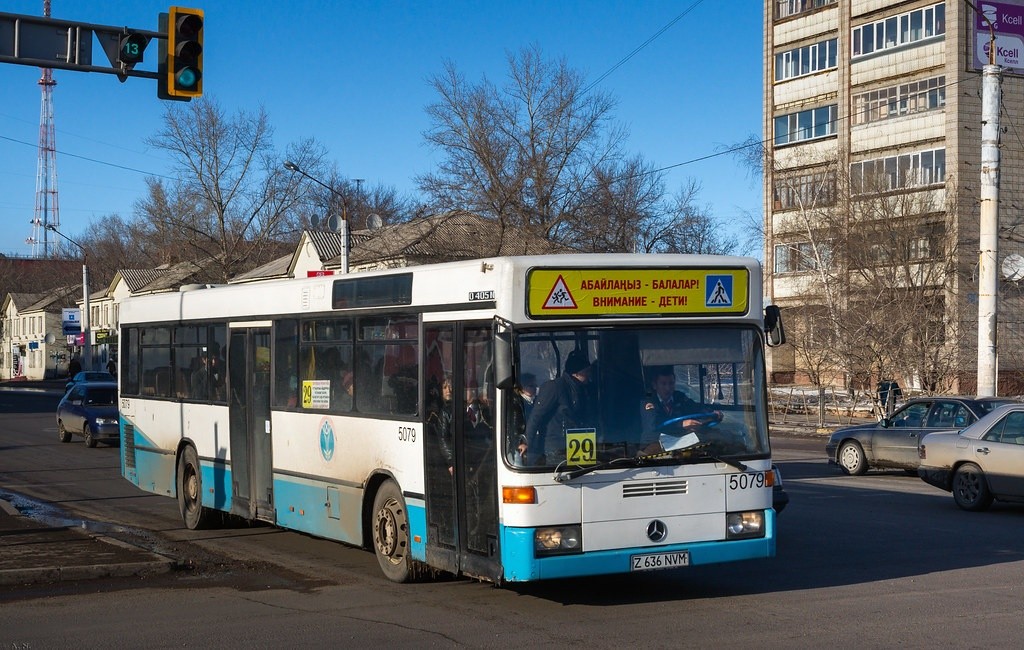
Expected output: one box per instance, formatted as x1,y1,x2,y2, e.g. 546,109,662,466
117,252,788,587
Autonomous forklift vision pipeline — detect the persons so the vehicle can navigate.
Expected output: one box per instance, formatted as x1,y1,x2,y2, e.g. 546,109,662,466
640,366,724,456
515,372,540,430
879,374,902,412
904,402,941,430
106,356,116,375
517,349,599,467
69,359,82,380
197,345,492,540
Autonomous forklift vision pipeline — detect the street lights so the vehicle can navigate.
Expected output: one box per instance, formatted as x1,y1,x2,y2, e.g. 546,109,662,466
283,162,349,273
45,224,94,376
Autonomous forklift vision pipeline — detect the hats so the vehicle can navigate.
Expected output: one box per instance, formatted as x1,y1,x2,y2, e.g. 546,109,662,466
565,349,591,374
343,372,360,390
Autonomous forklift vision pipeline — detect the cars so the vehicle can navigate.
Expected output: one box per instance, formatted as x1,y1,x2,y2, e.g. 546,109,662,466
825,397,1024,473
54,382,122,449
916,405,1024,512
64,372,116,394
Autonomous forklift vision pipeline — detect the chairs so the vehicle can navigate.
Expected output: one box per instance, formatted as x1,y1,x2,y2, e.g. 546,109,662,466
153,366,398,416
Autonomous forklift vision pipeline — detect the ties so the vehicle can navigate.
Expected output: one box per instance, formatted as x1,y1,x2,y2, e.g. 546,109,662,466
666,401,672,409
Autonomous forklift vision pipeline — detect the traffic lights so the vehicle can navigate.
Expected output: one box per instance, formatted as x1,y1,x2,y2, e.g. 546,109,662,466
167,5,205,99
119,32,148,65
